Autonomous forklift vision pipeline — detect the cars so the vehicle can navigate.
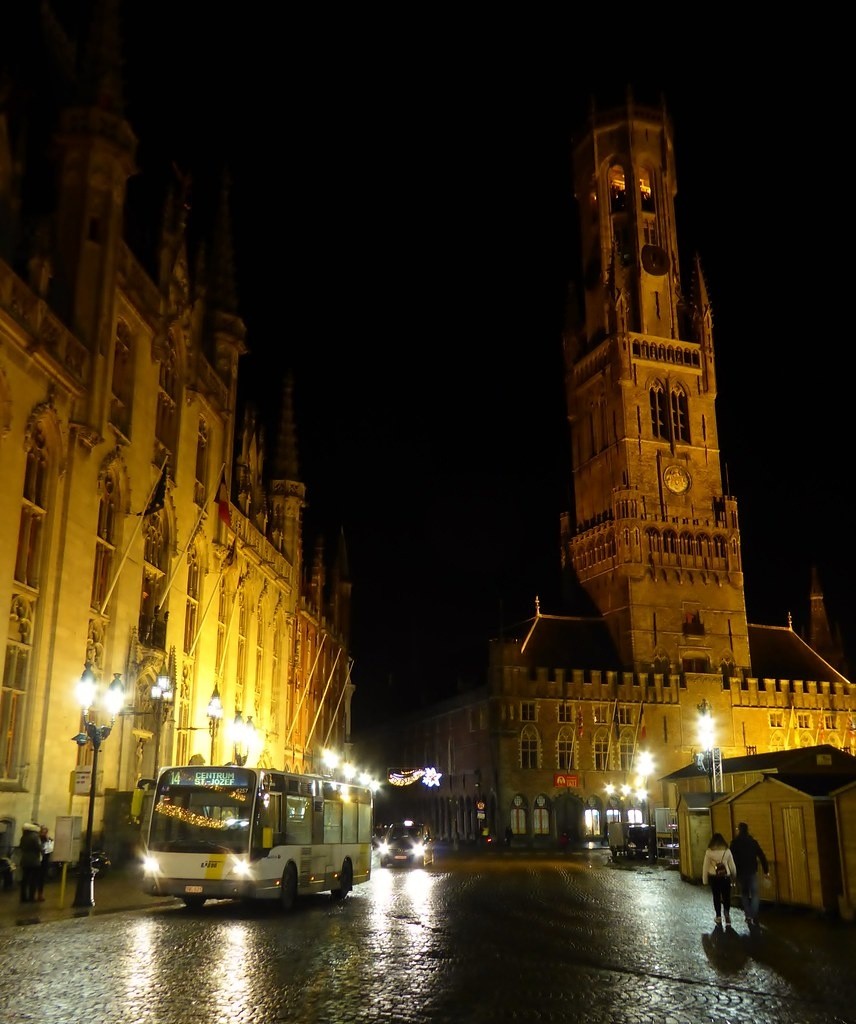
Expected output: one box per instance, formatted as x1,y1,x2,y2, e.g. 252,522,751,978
379,824,434,868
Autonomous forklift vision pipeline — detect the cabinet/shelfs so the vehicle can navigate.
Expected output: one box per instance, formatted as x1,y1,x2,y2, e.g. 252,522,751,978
655,832,679,862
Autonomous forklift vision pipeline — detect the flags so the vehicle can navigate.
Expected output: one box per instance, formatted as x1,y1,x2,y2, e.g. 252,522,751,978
614,704,620,740
792,712,800,742
847,714,853,738
638,703,646,738
818,715,824,740
214,473,232,527
577,704,584,737
137,465,167,516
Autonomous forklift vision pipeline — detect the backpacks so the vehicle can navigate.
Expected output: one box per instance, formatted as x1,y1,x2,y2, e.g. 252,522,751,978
710,847,728,877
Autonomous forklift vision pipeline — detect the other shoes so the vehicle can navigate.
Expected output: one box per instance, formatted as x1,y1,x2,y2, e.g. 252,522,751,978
753,918,759,924
714,917,721,923
745,918,751,924
726,918,731,925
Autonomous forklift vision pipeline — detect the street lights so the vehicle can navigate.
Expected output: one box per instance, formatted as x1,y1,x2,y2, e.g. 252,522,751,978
641,753,652,826
233,711,257,767
697,699,715,803
76,661,125,907
207,682,224,766
151,662,173,780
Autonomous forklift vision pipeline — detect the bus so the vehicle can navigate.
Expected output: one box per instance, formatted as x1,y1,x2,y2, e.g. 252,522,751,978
141,765,374,913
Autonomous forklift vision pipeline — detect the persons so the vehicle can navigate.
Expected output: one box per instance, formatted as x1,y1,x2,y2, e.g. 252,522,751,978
730,823,769,924
505,826,513,848
702,833,737,925
19,823,53,904
